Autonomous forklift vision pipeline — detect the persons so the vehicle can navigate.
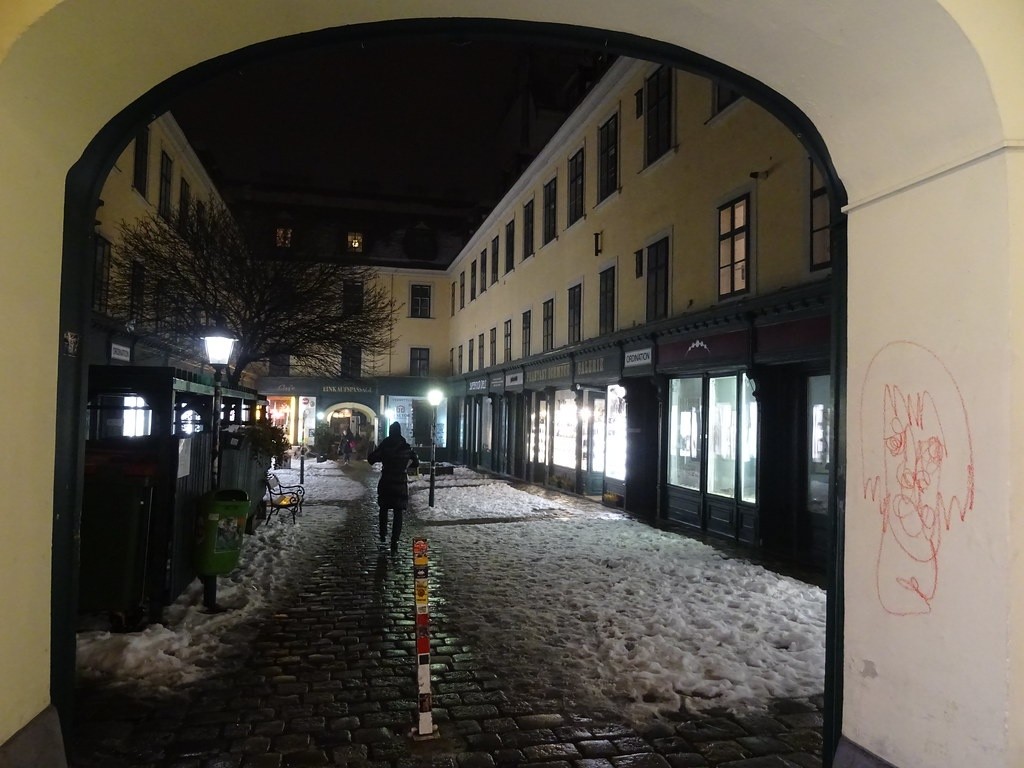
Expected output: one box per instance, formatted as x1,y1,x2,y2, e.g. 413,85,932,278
338,427,355,465
368,422,419,558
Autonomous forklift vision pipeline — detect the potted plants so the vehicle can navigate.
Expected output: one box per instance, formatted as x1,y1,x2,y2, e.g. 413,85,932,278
311,421,336,462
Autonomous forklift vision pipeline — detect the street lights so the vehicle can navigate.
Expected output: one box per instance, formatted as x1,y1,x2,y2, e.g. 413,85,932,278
200,316,241,490
428,388,445,509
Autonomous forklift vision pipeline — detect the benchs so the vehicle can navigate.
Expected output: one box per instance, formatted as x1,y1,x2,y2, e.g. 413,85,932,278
263,473,305,527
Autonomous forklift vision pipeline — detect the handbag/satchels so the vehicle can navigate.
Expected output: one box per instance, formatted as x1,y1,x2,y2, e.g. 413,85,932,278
349,438,356,447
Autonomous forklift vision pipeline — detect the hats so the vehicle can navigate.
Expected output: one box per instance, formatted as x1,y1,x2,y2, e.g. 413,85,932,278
389,421,401,435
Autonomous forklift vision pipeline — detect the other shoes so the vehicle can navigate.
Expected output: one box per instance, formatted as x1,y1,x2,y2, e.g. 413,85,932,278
379,531,387,535
345,462,348,464
391,536,399,541
